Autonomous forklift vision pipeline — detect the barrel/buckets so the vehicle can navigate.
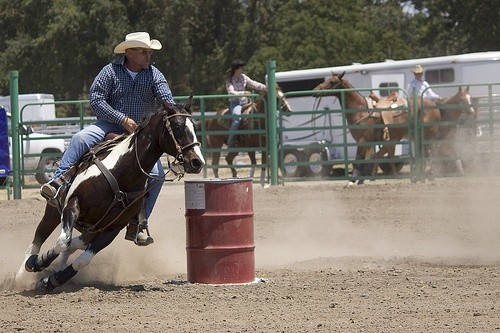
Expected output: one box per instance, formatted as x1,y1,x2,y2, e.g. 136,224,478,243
182,176,257,285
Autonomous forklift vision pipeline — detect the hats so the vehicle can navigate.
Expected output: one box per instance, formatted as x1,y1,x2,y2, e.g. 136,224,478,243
411,65,424,73
113,32,163,53
231,59,247,67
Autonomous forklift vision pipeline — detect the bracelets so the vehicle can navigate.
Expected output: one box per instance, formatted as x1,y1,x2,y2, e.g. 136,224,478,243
123,117,128,127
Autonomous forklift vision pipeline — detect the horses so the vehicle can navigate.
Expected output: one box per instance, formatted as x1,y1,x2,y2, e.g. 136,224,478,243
309,69,443,189
193,82,292,187
16,94,206,295
369,84,482,188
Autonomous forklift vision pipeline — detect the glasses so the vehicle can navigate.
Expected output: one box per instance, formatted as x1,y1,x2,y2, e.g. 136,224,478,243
133,48,154,55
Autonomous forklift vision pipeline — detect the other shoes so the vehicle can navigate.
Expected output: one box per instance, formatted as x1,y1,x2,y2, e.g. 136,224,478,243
41,182,60,207
125,223,154,244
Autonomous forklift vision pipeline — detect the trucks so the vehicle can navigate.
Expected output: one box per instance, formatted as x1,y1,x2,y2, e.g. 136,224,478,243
265,51,500,179
0,94,55,134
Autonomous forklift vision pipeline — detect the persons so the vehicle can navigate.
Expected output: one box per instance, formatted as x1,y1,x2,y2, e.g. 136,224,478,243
226,59,266,148
407,66,439,99
43,32,177,243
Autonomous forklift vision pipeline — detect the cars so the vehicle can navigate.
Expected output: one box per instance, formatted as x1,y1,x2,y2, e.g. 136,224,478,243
5,112,66,184
